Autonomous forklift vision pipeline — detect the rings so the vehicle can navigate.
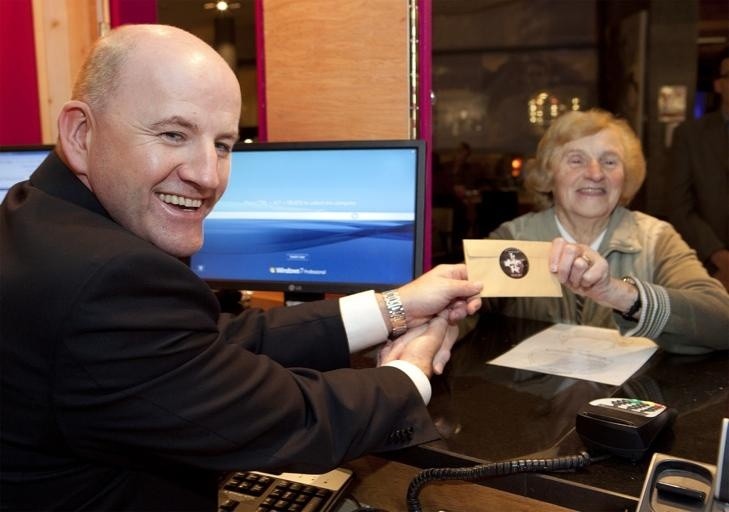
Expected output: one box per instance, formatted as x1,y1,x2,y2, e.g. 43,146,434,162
582,255,590,264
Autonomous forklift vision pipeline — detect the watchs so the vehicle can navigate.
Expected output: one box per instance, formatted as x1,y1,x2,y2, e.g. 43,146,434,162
612,277,642,324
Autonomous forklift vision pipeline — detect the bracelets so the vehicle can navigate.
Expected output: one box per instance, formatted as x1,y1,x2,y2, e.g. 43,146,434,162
382,289,408,338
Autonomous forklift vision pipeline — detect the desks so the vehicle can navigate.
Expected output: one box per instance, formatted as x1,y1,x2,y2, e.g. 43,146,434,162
179,313,729,510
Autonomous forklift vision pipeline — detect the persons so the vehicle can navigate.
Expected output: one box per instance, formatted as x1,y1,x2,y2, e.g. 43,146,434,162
432,161,471,260
0,23,484,511
665,46,729,282
376,108,729,366
445,142,478,188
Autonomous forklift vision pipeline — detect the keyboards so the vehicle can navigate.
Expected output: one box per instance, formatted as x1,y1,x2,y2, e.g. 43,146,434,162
219,463,358,510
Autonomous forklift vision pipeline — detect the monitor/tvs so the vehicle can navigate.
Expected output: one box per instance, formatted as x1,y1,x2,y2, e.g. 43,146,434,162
0,141,56,205
189,138,426,306
